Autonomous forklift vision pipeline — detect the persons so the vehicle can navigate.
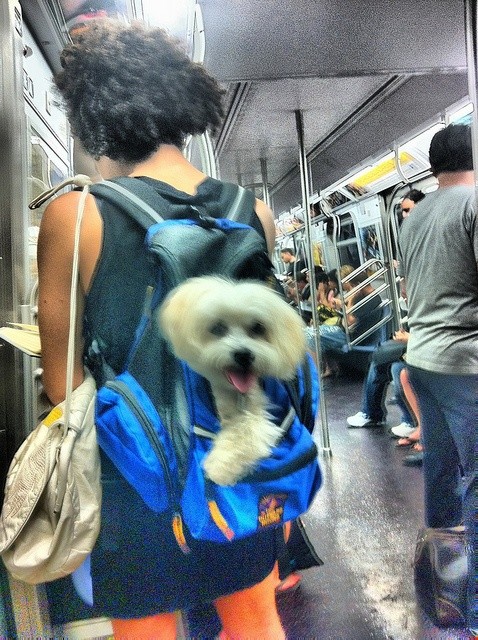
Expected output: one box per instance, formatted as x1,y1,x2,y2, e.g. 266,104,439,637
397,123,478,640
392,328,426,452
36,16,324,640
271,246,384,379
401,189,426,219
347,339,420,440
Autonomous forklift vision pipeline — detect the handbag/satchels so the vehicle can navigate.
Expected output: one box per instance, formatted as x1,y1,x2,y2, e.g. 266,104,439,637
414,526,469,629
372,342,406,366
0,365,102,585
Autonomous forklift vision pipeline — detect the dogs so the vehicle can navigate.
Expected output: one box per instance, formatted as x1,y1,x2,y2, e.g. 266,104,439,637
153,271,310,488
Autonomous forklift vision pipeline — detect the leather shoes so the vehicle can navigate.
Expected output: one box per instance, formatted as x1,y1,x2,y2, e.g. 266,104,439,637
402,452,422,466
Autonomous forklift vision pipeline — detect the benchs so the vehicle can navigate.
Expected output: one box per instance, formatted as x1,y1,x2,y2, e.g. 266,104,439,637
334,298,392,354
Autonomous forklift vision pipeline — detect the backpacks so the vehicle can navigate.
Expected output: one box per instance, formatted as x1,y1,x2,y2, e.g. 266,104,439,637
74,176,321,556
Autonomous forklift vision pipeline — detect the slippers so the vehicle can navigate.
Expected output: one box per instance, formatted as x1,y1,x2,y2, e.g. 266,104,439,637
406,443,425,455
394,437,419,447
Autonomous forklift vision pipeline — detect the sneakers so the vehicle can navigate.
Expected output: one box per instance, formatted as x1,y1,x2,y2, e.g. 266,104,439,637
321,368,331,377
346,411,382,427
388,422,416,437
